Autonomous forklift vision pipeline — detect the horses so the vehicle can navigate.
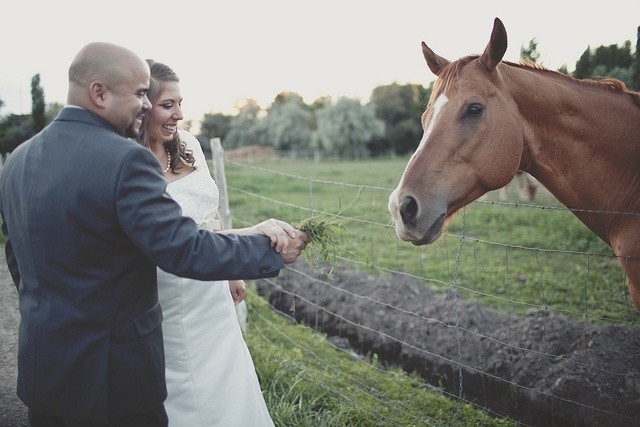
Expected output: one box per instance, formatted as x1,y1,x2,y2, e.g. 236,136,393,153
387,17,640,323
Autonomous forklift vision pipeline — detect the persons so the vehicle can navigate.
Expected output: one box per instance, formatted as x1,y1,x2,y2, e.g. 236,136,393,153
0,42,312,427
128,57,278,427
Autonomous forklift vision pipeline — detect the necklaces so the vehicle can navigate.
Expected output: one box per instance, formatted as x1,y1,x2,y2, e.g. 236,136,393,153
162,145,172,173
